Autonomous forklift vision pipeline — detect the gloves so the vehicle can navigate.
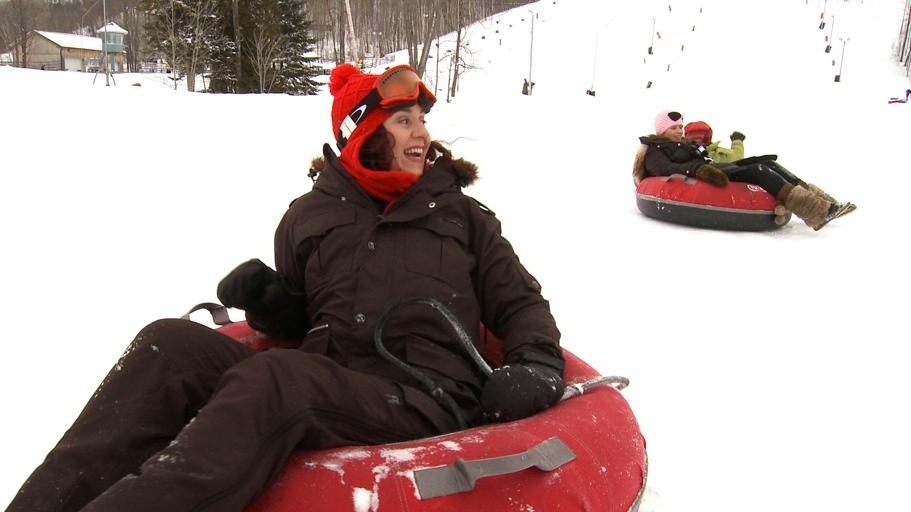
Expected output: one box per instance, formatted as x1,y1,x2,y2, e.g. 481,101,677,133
694,164,728,187
481,362,563,425
729,130,746,141
215,258,289,327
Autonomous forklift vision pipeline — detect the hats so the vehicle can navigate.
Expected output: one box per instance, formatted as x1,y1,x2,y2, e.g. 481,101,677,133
685,121,713,147
656,110,684,136
330,61,437,193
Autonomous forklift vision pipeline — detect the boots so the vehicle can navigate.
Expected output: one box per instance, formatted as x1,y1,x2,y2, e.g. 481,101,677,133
777,179,843,227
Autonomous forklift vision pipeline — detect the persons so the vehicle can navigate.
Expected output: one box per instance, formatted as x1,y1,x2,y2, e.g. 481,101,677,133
6,60,567,512
629,108,858,232
682,117,746,164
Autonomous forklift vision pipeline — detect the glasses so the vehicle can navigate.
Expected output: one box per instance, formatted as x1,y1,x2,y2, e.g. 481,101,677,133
336,64,436,151
